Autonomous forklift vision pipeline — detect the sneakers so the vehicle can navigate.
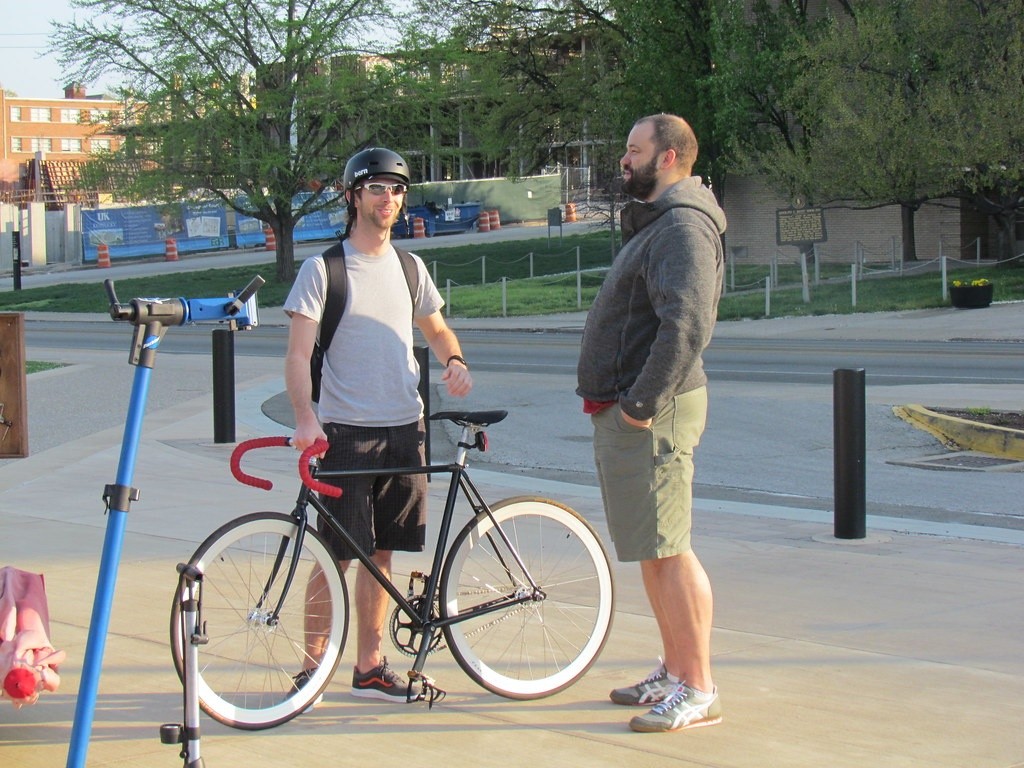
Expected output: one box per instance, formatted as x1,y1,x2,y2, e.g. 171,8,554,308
609,656,676,703
282,668,323,713
351,656,420,703
629,682,722,732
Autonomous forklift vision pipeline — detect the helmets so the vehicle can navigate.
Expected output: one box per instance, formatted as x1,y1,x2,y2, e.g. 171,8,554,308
343,148,410,205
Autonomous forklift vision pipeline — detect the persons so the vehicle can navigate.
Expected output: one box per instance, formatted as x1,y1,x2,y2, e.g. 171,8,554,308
579,112,728,737
279,148,474,713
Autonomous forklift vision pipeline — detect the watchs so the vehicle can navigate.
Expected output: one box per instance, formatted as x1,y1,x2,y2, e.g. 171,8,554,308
444,355,467,366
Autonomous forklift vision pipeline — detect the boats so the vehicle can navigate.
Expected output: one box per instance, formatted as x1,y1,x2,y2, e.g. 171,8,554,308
393,200,482,237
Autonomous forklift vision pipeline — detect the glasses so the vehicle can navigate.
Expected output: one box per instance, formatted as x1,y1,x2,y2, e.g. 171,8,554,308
354,183,408,196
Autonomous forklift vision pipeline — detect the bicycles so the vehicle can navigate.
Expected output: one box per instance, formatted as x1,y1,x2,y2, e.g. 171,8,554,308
170,410,614,732
